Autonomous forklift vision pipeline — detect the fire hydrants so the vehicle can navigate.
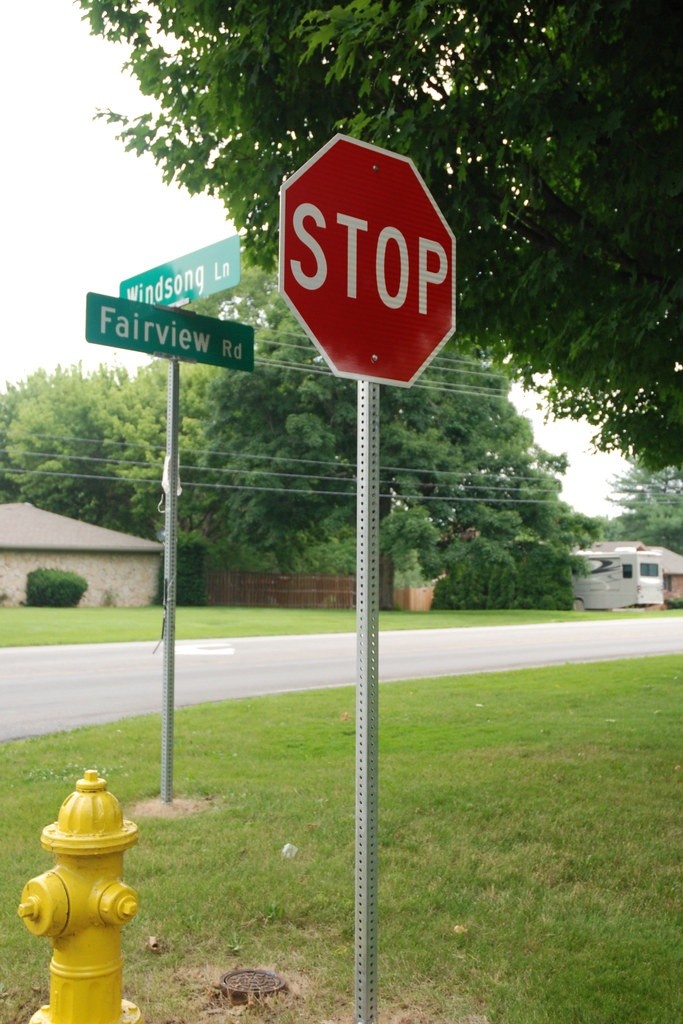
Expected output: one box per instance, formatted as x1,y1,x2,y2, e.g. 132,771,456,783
16,770,145,1024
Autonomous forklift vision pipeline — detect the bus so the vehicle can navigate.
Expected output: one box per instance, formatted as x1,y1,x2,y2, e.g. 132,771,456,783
570,547,667,611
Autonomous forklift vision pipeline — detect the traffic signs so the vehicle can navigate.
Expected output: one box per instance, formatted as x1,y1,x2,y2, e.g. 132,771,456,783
84,292,256,372
120,234,240,308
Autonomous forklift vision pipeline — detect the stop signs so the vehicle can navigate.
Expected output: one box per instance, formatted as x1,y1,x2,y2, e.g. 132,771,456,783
276,133,458,389
